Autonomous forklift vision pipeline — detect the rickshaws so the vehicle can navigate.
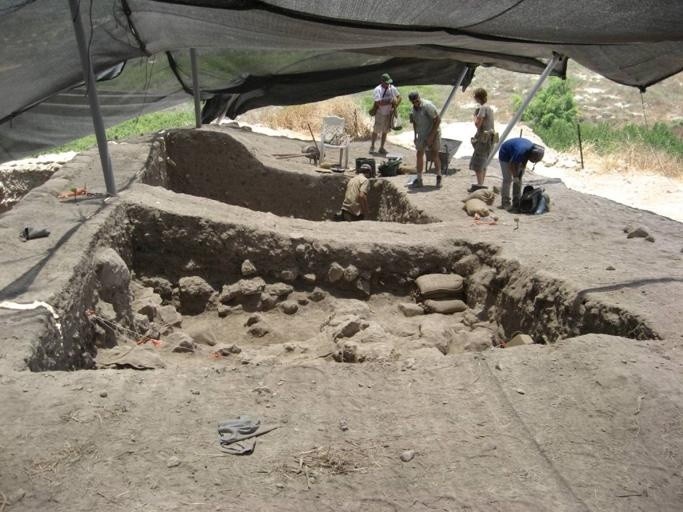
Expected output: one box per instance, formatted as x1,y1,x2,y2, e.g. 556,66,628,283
424,137,463,172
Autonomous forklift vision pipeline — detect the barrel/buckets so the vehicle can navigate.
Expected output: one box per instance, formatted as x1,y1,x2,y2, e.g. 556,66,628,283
356,157,375,176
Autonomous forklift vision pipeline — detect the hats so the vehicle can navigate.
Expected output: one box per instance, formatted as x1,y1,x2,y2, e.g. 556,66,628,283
409,92,419,101
360,163,372,175
382,73,393,84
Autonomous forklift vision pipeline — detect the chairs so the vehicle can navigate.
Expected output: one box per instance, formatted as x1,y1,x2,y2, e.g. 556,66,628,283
319,115,348,169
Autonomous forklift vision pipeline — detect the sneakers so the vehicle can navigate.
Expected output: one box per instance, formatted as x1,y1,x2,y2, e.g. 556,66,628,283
408,179,423,188
379,148,388,153
436,178,442,187
468,184,482,193
369,146,374,153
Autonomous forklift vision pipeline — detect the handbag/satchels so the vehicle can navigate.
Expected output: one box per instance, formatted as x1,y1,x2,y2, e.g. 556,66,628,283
391,114,402,130
368,103,378,115
521,186,550,214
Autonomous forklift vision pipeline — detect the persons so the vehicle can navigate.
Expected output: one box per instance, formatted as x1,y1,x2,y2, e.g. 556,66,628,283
406,90,442,188
369,73,402,154
498,137,545,211
469,87,495,184
341,163,374,220
